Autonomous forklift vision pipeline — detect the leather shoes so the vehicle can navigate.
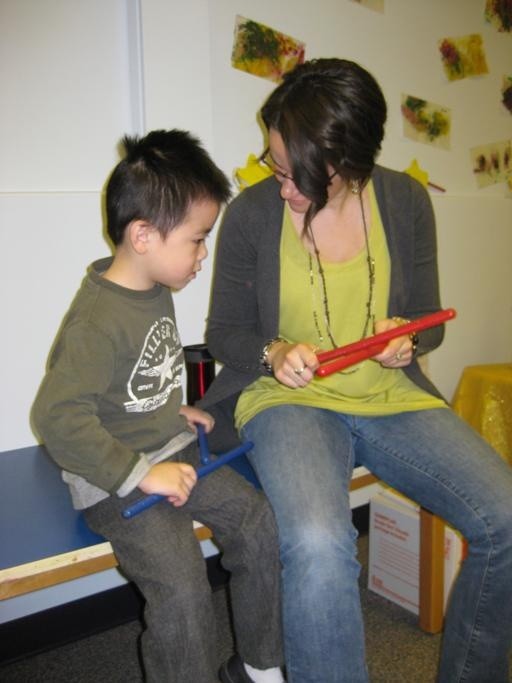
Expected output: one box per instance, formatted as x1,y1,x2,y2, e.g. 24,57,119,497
218,653,255,683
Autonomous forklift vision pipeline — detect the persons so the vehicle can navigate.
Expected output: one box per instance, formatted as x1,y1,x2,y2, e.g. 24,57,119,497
29,128,286,682
193,57,512,681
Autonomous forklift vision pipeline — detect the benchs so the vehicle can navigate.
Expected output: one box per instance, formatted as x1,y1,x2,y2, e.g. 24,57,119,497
0,443,380,601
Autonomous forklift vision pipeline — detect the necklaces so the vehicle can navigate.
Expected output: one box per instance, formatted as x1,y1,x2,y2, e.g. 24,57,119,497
303,194,380,377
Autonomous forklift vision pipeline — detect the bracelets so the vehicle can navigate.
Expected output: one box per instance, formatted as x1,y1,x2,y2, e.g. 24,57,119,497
392,315,418,356
259,337,288,372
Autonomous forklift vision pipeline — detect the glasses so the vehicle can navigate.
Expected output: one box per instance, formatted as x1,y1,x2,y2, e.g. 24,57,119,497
258,146,338,185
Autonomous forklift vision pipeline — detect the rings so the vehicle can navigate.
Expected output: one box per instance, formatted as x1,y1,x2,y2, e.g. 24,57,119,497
294,364,308,375
394,351,404,360
